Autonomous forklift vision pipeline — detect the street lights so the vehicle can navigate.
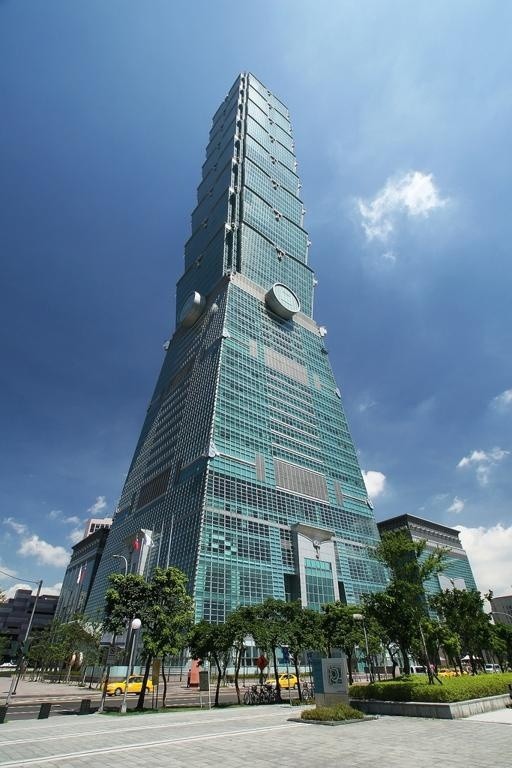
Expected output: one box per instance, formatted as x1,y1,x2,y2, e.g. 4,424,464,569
119,618,142,712
488,611,512,618
353,614,373,683
113,554,128,576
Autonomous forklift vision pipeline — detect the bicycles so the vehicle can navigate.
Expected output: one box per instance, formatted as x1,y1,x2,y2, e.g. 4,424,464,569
302,682,315,698
243,685,281,704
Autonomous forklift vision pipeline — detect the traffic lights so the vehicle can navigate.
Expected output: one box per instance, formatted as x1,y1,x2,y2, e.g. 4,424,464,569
19,639,29,659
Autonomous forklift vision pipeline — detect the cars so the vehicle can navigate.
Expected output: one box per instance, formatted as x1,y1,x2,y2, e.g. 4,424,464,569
106,675,155,696
437,668,468,677
264,673,300,689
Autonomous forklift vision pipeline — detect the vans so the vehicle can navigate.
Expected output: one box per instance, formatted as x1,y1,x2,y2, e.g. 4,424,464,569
485,664,500,674
408,666,428,674
